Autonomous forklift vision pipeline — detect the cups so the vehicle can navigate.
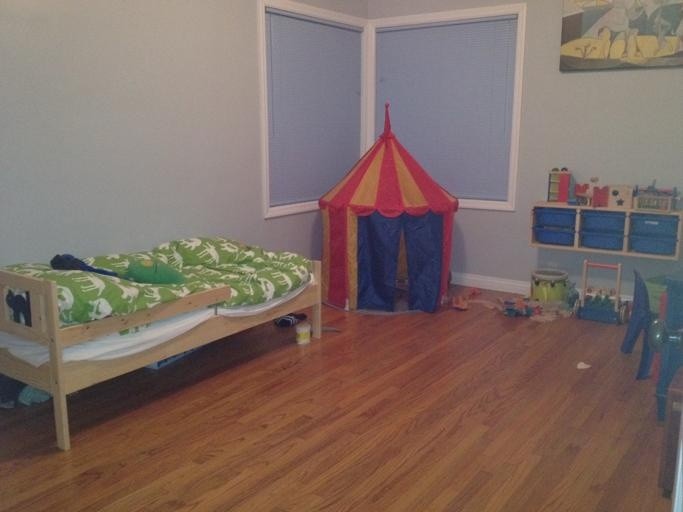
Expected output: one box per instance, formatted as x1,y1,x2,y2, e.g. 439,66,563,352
295,324,311,345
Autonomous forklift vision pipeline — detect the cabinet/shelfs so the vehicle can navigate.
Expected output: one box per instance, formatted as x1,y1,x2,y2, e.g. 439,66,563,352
528,201,683,261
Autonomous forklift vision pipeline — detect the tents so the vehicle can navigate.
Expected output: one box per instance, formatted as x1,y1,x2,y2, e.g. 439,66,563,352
319,100,462,314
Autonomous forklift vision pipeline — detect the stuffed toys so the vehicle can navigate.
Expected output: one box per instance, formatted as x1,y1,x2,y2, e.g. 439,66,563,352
125,255,187,283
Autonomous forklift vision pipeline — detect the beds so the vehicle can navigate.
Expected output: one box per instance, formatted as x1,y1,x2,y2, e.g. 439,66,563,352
1,236,321,452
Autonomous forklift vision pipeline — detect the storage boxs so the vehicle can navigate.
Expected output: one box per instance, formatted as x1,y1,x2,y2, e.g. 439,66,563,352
532,207,679,257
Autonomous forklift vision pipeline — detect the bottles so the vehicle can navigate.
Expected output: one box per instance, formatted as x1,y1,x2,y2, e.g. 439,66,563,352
533,299,540,315
